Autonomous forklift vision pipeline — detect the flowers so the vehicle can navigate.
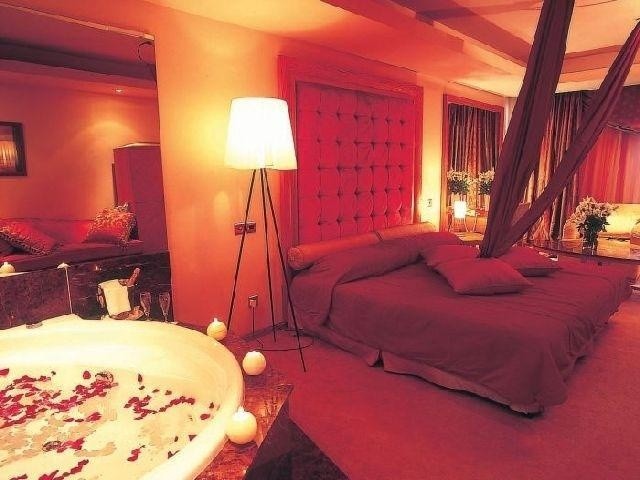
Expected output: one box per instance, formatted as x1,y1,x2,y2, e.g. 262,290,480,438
445,170,475,197
572,197,619,250
480,167,496,197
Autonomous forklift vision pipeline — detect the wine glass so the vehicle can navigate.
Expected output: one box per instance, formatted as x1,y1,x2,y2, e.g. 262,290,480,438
158,291,171,323
139,291,152,322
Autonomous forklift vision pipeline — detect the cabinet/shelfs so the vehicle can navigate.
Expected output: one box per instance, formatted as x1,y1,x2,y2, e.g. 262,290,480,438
112,141,168,252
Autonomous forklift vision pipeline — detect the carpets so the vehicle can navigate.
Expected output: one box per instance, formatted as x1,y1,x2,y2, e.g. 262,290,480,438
243,303,640,479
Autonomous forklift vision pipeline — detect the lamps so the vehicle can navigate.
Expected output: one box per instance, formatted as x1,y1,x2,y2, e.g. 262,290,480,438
221,97,307,375
452,202,469,220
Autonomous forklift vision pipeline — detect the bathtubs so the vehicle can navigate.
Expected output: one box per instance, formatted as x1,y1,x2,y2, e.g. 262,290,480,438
0,316,246,480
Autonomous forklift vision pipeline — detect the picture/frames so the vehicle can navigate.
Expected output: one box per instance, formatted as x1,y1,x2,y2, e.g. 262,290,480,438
0,121,27,178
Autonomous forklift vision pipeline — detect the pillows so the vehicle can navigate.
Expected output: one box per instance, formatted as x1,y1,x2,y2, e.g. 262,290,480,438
1,220,58,256
83,208,137,249
436,256,534,294
421,244,480,269
498,245,562,279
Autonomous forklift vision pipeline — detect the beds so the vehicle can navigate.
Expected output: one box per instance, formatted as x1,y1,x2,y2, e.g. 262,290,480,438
284,224,635,414
